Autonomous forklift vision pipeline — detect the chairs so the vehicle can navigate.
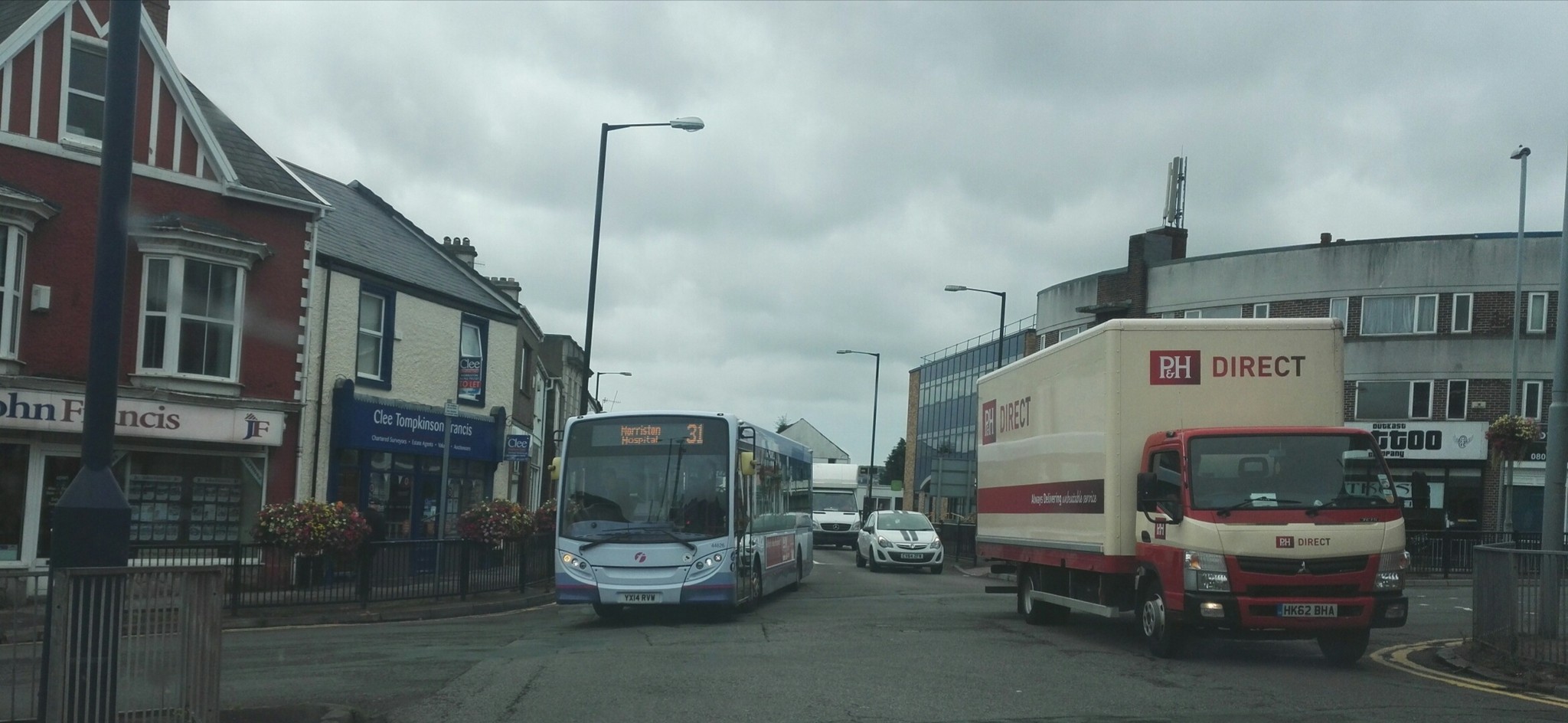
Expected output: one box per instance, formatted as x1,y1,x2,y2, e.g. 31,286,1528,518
1302,449,1347,495
1237,457,1271,493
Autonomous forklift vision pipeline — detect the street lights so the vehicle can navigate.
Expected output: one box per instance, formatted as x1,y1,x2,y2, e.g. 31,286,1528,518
579,115,707,414
836,349,880,515
595,372,633,402
1503,144,1532,542
943,284,1007,369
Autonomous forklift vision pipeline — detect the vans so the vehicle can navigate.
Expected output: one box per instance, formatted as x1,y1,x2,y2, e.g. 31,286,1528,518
811,463,865,551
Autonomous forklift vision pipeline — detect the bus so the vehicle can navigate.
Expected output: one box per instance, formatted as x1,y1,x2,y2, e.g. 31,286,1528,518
547,411,815,619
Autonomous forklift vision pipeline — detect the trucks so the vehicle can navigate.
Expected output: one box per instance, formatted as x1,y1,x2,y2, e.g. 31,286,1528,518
974,317,1413,665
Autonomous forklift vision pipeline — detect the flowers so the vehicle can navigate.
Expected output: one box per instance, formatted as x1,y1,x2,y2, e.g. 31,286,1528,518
249,496,372,554
454,497,582,546
1485,414,1540,474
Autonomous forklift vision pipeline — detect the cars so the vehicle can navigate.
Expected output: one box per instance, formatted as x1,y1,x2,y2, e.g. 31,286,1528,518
855,510,945,575
